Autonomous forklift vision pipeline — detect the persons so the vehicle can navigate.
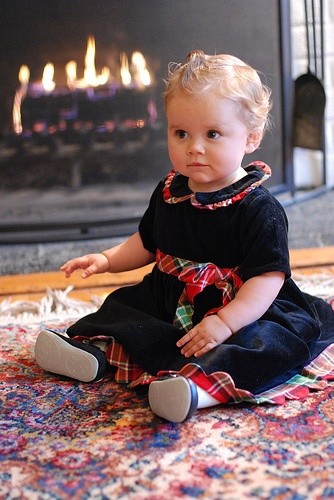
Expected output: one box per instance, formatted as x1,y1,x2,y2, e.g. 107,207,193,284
32,50,333,423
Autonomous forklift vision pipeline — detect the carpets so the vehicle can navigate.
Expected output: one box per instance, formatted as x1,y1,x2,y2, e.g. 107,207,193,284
0,271,334,500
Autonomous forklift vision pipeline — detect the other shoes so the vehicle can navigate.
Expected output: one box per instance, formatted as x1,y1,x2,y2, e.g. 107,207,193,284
149,376,197,424
35,329,107,383
